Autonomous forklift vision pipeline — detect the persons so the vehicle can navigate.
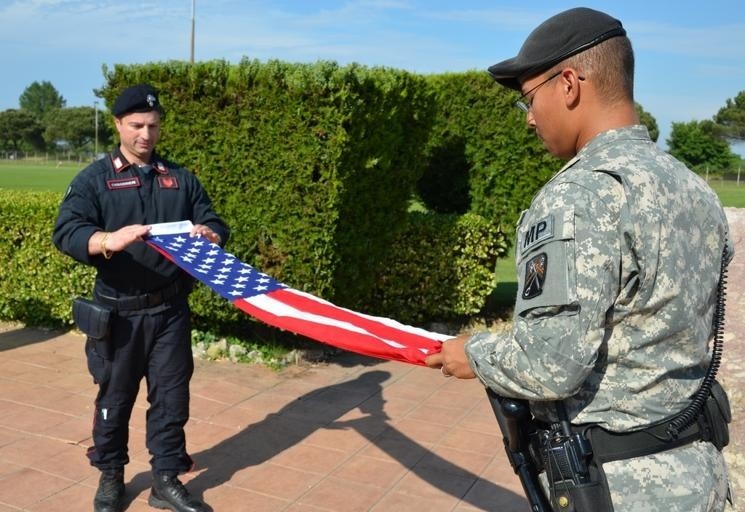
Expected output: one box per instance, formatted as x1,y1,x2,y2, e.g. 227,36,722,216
52,84,230,512
425,7,734,512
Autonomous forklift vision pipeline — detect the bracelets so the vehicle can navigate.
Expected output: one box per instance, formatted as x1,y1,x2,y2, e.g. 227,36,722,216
100,232,113,260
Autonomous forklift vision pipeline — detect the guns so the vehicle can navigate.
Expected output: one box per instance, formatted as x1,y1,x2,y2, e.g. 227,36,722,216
485,387,614,512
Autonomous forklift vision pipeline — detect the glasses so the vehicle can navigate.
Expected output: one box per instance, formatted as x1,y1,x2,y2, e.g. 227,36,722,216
512,70,586,115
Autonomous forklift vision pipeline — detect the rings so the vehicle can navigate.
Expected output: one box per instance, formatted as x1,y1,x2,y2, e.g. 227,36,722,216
441,367,450,377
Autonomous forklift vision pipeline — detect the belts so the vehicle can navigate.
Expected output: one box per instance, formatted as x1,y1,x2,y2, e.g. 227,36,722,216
92,283,181,311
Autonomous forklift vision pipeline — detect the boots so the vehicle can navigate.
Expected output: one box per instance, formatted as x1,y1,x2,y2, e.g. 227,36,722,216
94,470,125,511
147,471,214,511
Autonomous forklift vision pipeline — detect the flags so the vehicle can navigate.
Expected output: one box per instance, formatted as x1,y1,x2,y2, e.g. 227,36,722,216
140,220,451,368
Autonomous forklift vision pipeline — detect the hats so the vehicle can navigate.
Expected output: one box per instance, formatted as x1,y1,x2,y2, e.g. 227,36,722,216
489,7,627,89
111,85,160,117
